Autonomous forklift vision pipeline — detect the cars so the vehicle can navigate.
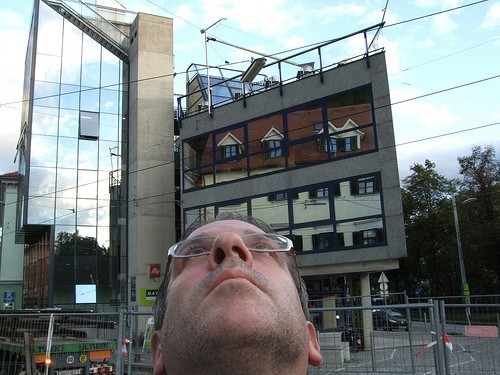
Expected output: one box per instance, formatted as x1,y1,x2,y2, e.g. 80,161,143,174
373,309,411,330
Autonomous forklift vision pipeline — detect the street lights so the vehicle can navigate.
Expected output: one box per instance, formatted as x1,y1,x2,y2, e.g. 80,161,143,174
452,192,478,315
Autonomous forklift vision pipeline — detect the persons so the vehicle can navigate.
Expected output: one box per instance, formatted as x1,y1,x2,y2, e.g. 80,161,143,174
149,212,322,375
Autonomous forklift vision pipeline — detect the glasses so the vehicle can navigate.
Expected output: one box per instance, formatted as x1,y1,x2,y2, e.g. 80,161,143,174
157,234,307,327
